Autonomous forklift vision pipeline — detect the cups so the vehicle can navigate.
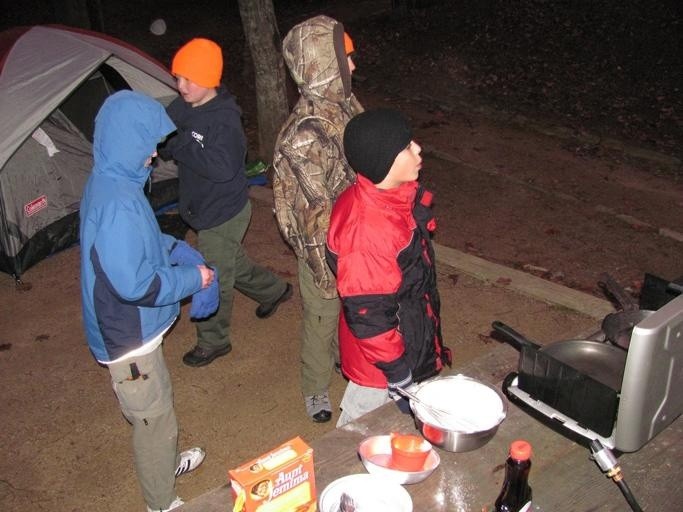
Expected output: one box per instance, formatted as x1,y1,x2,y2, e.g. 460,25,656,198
390,434,432,472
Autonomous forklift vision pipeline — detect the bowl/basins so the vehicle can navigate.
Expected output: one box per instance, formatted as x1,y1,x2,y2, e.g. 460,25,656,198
357,434,441,484
409,373,508,453
319,474,413,512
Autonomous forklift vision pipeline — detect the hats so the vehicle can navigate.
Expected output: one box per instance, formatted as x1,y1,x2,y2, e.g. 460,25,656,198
344,31,355,55
171,38,224,88
343,107,417,184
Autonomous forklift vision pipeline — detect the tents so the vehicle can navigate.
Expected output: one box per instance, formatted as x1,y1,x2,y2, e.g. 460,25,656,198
1,16,180,283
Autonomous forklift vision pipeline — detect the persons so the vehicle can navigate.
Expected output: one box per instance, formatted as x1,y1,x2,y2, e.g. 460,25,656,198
79,89,206,511
273,14,365,424
158,37,294,368
325,106,452,425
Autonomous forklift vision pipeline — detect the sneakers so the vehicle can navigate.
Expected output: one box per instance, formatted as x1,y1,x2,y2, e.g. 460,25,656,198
304,390,332,422
174,447,205,478
182,343,232,367
146,496,184,512
255,283,293,319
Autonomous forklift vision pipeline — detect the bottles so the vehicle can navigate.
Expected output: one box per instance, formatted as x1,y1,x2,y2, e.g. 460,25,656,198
494,440,533,512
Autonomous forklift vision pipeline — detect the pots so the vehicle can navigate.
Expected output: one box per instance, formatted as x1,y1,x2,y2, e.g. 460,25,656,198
599,271,655,349
492,320,628,394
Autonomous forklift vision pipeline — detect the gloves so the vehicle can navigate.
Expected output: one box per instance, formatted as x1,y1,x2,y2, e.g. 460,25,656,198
387,368,414,414
